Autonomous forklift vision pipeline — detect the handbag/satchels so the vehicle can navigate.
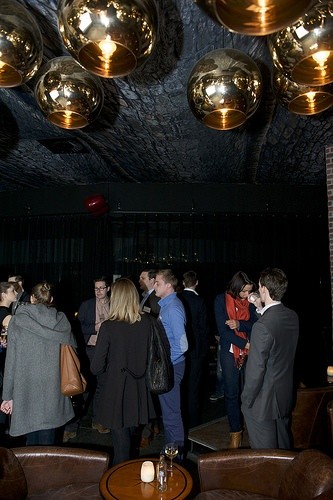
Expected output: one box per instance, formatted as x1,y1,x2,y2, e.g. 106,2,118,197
146,319,175,395
60,343,87,398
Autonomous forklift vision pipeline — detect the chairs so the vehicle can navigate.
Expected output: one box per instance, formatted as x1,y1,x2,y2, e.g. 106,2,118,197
195,449,333,500
0,446,109,500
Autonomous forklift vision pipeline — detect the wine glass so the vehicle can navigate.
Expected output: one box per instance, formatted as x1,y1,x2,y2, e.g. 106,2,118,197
156,460,168,493
164,443,178,471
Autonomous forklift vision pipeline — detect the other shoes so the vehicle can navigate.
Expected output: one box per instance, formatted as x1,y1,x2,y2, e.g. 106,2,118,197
62,431,76,443
91,421,110,434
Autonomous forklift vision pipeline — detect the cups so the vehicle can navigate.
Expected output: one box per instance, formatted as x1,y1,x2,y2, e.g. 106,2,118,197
140,460,155,483
1,326,8,346
248,293,260,303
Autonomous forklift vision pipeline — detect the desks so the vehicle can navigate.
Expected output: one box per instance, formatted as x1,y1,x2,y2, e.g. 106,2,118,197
100,458,194,500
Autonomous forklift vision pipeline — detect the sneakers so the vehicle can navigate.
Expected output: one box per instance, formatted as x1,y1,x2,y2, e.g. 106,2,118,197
209,391,225,401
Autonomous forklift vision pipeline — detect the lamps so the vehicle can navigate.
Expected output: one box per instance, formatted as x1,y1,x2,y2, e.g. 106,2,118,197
265,0,333,87
56,0,160,79
0,0,43,86
35,55,104,130
186,27,262,130
273,68,333,115
193,0,317,37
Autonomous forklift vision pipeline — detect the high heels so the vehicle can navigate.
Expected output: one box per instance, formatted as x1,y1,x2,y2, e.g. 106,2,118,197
230,430,243,449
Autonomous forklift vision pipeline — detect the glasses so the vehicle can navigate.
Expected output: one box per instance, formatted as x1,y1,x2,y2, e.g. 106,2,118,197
94,286,107,291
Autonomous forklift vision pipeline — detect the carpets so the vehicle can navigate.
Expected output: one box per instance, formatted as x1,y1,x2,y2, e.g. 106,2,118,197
187,384,321,453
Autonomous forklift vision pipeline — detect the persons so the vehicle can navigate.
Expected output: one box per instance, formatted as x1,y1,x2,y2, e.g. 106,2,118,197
0,275,76,446
139,268,188,462
178,270,208,429
79,277,110,433
209,271,258,447
241,267,299,449
93,278,166,464
